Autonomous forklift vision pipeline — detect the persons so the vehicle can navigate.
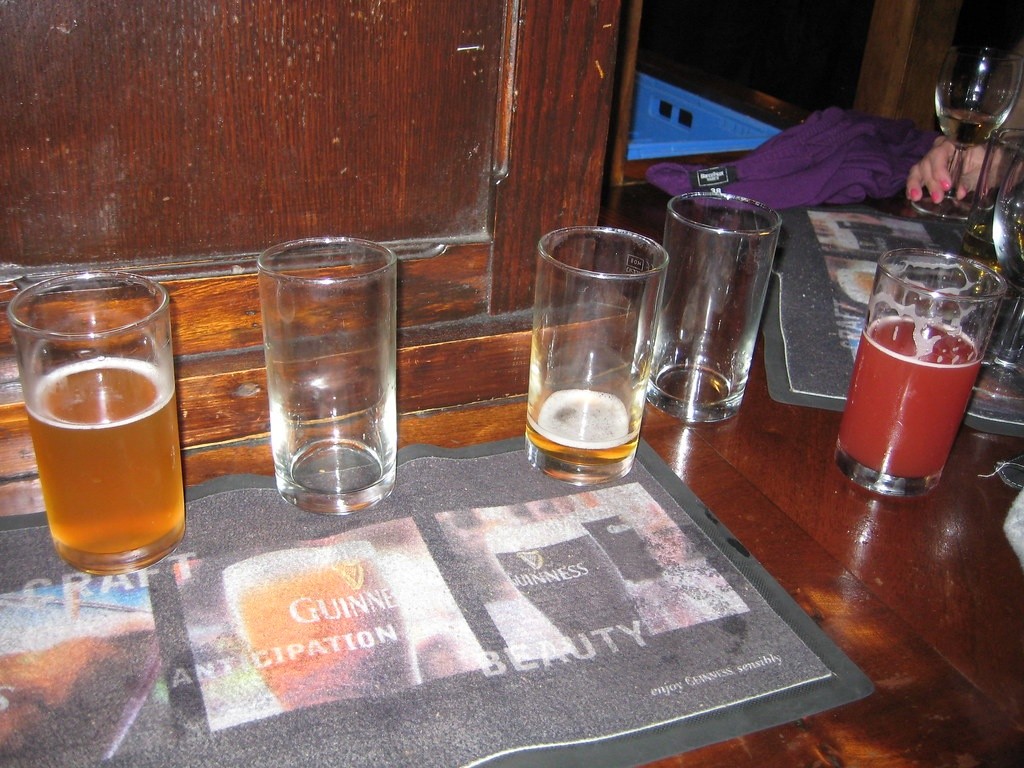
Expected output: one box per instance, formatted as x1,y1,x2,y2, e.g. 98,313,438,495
906,40,1024,205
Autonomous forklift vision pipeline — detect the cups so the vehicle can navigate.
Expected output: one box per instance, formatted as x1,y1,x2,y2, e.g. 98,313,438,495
257,237,397,516
833,248,1007,497
525,193,783,486
7,272,186,575
961,128,1024,258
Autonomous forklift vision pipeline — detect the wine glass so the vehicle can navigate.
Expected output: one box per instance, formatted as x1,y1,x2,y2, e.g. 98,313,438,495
973,144,1023,400
911,44,1024,220
